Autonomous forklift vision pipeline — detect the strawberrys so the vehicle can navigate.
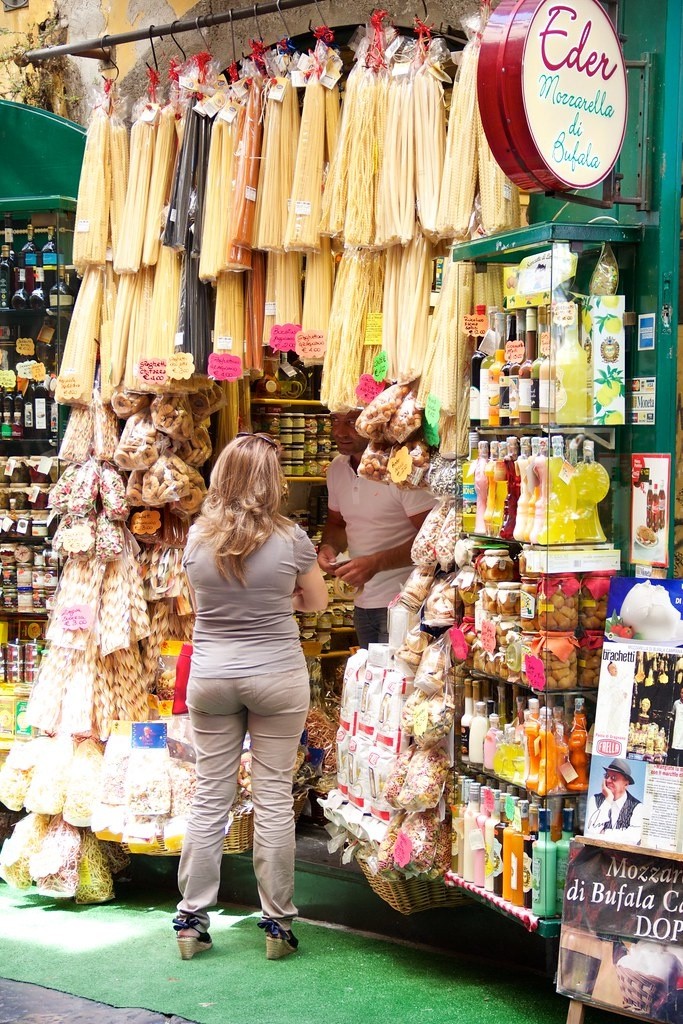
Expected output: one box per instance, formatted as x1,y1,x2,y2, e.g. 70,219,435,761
610,623,633,639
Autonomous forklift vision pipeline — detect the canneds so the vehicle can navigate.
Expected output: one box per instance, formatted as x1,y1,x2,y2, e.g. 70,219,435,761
249,411,340,478
455,538,617,693
283,509,356,654
0,456,59,684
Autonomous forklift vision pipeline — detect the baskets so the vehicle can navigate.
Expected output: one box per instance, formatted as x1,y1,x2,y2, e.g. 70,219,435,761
118,787,309,856
307,791,332,826
348,841,478,915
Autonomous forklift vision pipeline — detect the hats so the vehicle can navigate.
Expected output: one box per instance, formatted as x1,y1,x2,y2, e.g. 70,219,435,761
603,758,635,785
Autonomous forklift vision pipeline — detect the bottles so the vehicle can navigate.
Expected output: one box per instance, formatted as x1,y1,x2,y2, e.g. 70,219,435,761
450,774,577,919
461,432,612,544
0,214,76,309
644,480,666,532
471,302,588,424
459,678,592,795
0,370,61,439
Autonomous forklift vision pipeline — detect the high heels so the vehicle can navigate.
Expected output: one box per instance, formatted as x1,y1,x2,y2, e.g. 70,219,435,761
257,921,299,959
173,918,213,960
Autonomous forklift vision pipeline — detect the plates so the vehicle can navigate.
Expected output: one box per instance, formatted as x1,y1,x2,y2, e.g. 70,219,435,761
634,536,659,549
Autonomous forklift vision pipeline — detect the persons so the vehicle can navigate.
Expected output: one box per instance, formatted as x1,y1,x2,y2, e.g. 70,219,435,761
585,759,644,845
668,687,683,767
173,434,327,958
606,661,628,725
317,410,441,651
634,467,650,494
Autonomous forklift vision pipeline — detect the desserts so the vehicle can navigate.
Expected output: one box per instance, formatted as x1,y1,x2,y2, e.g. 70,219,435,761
620,580,681,641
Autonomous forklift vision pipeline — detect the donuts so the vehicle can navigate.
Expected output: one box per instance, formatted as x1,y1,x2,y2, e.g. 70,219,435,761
110,373,224,516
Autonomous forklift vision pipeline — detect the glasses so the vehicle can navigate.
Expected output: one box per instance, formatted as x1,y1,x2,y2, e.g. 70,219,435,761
236,431,278,448
603,773,617,781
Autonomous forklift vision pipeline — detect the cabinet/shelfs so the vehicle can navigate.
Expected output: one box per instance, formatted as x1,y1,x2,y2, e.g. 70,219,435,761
317,626,357,661
0,194,84,766
248,398,329,481
444,216,662,939
